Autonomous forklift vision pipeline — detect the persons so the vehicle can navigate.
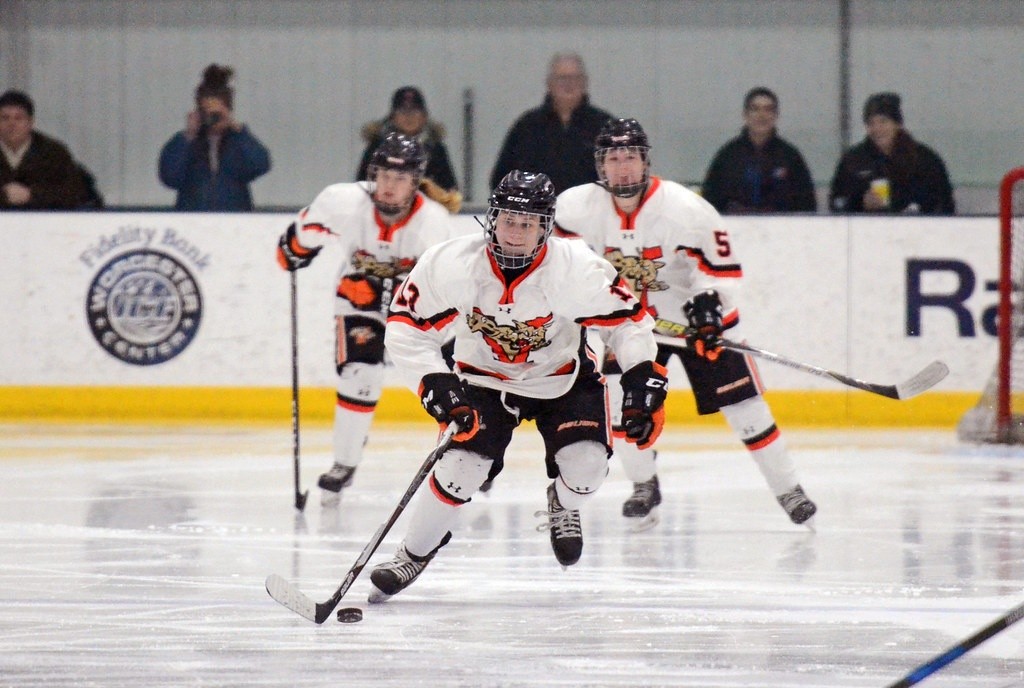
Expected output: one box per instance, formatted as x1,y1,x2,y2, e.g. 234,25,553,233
827,92,956,216
547,115,821,534
354,86,460,194
700,85,819,217
366,168,668,606
490,48,622,200
276,130,466,507
158,62,272,212
0,87,76,213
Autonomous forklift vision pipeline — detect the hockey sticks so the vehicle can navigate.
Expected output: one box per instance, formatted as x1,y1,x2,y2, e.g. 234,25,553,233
651,317,953,401
889,604,1024,687
461,84,476,206
288,269,311,513
262,419,462,625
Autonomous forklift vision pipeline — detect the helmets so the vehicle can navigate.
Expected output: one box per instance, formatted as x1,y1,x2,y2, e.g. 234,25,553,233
593,118,652,198
366,132,427,215
484,170,556,269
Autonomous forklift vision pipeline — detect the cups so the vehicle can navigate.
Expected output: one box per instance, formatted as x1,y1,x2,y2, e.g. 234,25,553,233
871,178,890,211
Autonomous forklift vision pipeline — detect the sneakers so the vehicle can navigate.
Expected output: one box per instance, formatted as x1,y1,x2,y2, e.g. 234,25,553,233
623,474,662,533
318,462,358,505
776,484,817,534
533,480,583,570
367,530,451,604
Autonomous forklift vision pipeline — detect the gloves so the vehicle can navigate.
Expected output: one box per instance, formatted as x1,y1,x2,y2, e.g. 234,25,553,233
611,360,669,450
418,372,481,441
336,273,403,318
277,222,322,271
682,290,724,361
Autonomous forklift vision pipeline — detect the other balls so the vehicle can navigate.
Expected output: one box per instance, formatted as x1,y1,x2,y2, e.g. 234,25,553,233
336,608,364,623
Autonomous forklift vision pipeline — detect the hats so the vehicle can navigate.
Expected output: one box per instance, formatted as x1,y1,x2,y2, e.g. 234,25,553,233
863,92,903,124
391,88,424,106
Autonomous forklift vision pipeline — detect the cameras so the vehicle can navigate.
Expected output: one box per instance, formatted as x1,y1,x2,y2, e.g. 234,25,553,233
199,111,219,128
